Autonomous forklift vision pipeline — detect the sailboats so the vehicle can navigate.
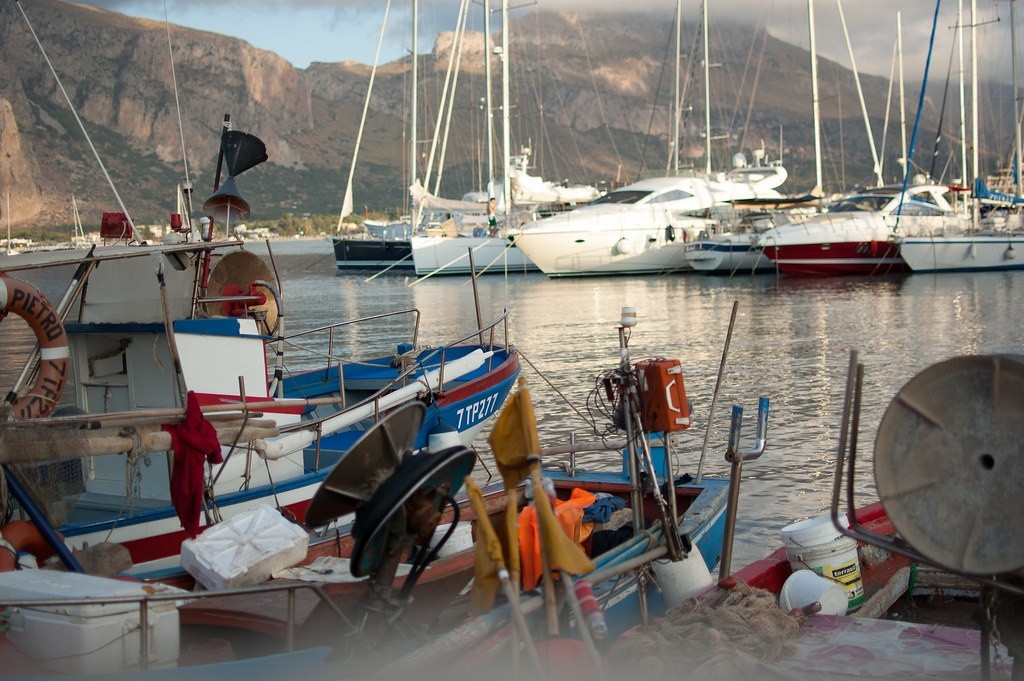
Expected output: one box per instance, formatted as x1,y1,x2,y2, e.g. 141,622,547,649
330,0,1024,277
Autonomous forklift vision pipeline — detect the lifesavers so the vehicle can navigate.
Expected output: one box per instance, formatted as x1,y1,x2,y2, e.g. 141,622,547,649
0,273,69,419
0,516,61,571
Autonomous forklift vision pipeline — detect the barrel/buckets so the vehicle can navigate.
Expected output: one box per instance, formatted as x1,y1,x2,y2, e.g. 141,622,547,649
779,569,849,616
780,511,850,548
783,530,866,612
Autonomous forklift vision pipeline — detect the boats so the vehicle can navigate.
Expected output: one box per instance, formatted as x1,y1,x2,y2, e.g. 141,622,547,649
0,305,731,681
0,110,524,582
58,465,732,681
587,349,1024,681
